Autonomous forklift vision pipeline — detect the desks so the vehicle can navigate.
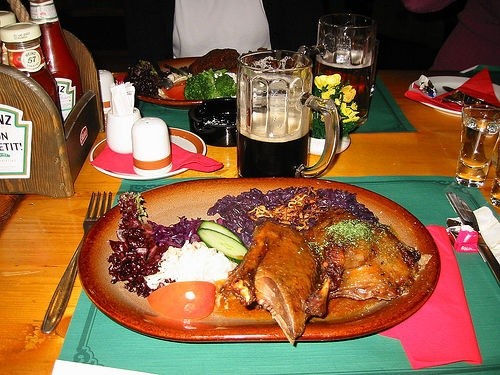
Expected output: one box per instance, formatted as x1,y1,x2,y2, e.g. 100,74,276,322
0,70,500,375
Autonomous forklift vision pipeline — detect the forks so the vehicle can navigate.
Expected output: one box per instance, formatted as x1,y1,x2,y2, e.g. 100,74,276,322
41,191,112,334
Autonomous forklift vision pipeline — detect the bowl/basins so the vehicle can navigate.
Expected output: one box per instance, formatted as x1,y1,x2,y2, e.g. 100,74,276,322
189,97,237,147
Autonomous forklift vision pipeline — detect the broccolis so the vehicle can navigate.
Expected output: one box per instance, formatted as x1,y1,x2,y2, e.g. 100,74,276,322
184,68,236,100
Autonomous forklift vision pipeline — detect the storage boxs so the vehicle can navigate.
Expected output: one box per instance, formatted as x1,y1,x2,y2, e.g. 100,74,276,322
0,30,106,198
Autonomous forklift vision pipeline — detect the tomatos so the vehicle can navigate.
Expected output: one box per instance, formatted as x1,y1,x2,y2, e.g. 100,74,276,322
163,80,187,100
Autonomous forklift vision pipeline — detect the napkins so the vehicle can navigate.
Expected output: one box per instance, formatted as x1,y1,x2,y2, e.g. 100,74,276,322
90,141,224,173
404,67,500,112
447,206,500,262
377,225,481,370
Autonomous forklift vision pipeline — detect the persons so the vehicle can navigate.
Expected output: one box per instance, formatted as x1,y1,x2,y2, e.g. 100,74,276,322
124,0,500,73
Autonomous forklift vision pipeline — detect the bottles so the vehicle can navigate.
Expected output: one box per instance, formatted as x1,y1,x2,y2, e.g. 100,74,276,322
0,0,82,122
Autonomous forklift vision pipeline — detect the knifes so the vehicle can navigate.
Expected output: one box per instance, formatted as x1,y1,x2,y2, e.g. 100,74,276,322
443,86,500,111
445,191,500,285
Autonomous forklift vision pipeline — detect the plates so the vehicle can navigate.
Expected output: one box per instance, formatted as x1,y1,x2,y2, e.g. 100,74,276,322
409,75,500,117
114,58,204,106
77,177,441,340
90,127,206,181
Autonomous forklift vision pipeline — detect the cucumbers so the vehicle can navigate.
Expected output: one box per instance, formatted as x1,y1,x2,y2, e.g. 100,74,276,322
196,229,247,263
200,222,244,245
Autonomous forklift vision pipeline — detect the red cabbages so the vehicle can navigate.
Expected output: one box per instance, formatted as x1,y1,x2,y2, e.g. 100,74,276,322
147,186,379,248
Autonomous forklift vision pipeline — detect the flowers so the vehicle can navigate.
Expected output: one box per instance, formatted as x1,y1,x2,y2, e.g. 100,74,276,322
311,74,360,139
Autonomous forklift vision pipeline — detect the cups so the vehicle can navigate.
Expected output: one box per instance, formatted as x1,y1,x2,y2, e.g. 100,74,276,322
98,68,135,114
235,49,340,178
106,107,141,154
132,117,172,176
456,105,500,206
316,14,376,123
